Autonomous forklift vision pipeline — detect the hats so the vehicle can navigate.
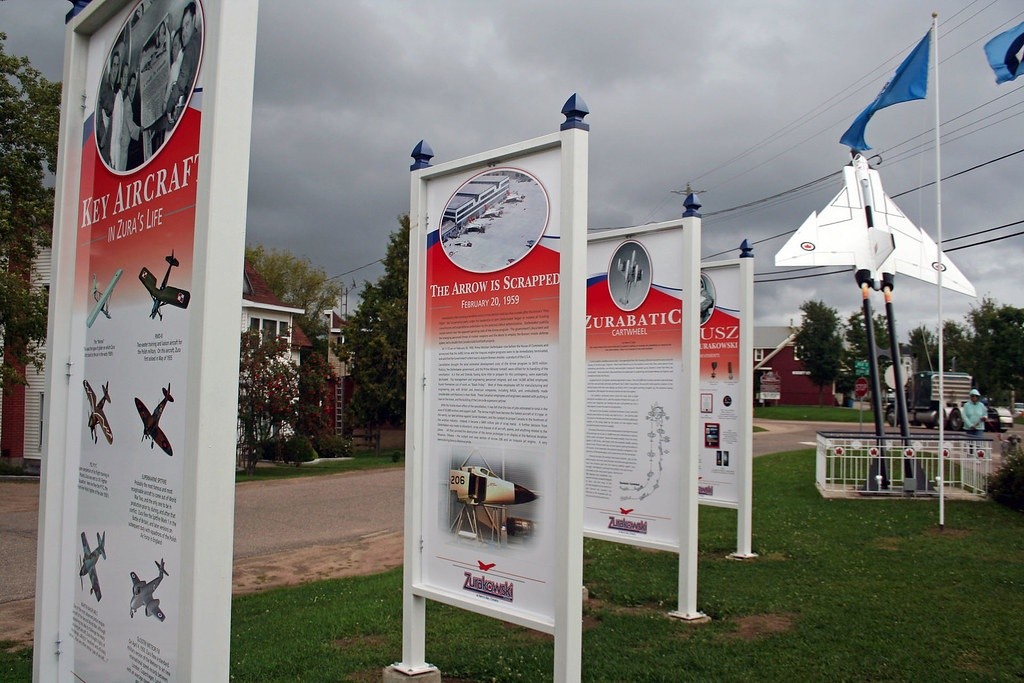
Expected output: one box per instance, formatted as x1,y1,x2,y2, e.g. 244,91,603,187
970,389,980,397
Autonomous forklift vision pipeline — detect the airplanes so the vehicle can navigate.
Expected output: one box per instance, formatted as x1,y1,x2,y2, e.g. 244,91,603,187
77,531,106,602
85,267,125,329
129,557,169,624
83,379,114,446
134,382,175,457
120,204,191,321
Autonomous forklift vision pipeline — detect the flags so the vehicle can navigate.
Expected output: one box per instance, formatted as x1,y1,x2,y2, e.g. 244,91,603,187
983,22,1024,84
838,26,929,152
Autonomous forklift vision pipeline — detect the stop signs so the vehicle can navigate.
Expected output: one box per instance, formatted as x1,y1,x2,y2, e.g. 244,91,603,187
853,377,869,397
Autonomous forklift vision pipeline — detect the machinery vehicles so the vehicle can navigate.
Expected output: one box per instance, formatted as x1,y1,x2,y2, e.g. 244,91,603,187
885,371,1014,433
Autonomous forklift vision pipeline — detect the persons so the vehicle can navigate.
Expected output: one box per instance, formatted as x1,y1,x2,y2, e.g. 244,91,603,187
163,27,184,120
96,52,120,166
120,73,145,170
167,3,200,124
959,388,988,456
155,23,168,55
111,62,130,171
